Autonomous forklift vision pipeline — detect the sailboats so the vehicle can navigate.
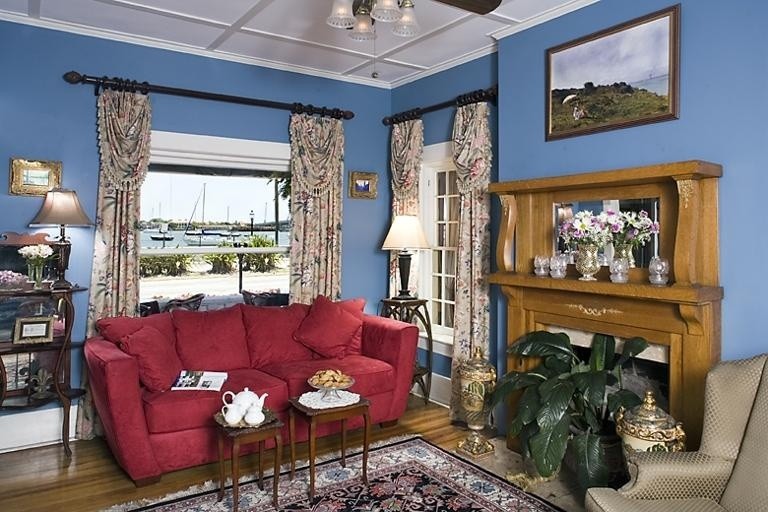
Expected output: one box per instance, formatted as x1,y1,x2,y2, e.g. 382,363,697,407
183,182,250,246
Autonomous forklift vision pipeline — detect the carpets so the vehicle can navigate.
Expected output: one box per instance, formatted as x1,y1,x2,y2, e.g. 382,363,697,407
91,429,573,511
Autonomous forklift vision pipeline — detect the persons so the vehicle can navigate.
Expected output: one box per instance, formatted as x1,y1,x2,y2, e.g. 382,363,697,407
572,102,587,121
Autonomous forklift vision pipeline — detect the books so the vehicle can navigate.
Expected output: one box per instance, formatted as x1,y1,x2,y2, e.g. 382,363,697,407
170,369,228,392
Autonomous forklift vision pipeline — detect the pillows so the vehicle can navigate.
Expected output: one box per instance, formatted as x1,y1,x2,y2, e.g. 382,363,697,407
119,321,184,393
171,300,252,374
239,300,315,372
94,311,183,384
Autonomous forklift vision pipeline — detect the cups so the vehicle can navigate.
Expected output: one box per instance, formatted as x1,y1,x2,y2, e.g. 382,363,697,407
648,257,670,284
609,258,630,284
533,255,568,279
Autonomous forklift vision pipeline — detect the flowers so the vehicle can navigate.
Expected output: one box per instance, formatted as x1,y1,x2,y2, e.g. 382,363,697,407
597,206,662,250
16,243,53,264
553,205,608,249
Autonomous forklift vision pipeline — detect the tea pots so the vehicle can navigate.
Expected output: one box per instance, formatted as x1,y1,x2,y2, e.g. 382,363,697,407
222,386,268,414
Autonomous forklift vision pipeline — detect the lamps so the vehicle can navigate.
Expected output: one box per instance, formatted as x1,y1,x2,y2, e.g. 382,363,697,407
323,1,421,79
26,186,95,289
378,213,432,300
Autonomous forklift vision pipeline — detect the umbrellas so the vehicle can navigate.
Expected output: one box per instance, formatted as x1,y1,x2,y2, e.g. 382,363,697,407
562,94,577,105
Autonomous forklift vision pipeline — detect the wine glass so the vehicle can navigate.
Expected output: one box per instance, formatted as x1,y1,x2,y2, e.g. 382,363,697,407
307,375,355,402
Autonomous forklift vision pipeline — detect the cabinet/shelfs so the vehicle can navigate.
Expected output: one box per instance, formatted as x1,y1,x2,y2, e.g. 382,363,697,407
379,295,433,407
0,234,90,460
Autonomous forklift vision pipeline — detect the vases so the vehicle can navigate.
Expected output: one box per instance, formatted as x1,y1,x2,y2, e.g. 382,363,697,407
607,241,632,284
625,243,635,269
574,241,603,284
26,260,47,290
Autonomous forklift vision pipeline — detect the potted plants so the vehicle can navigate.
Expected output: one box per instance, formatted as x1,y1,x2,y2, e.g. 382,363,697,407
476,322,656,511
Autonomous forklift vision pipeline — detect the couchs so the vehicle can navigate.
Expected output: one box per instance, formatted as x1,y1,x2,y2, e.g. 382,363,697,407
581,353,768,512
79,294,421,492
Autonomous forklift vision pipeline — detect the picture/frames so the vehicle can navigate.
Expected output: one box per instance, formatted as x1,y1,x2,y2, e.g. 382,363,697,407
346,170,379,200
5,153,63,200
543,2,685,143
11,311,53,347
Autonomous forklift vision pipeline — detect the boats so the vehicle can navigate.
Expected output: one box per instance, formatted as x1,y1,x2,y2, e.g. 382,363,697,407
142,200,175,240
254,197,290,231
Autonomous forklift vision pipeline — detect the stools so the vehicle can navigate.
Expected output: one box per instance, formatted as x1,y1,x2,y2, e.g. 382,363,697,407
210,406,286,512
284,386,374,505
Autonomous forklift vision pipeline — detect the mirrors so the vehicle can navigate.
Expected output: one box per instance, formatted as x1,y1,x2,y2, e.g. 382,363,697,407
551,195,662,269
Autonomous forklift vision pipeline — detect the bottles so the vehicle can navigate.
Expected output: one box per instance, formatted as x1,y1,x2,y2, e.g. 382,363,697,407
221,404,266,425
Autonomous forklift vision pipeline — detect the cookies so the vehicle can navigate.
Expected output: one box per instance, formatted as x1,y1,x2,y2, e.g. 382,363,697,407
309,368,351,388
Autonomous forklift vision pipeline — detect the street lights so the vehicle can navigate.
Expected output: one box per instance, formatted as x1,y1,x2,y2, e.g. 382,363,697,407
250,209,254,235
